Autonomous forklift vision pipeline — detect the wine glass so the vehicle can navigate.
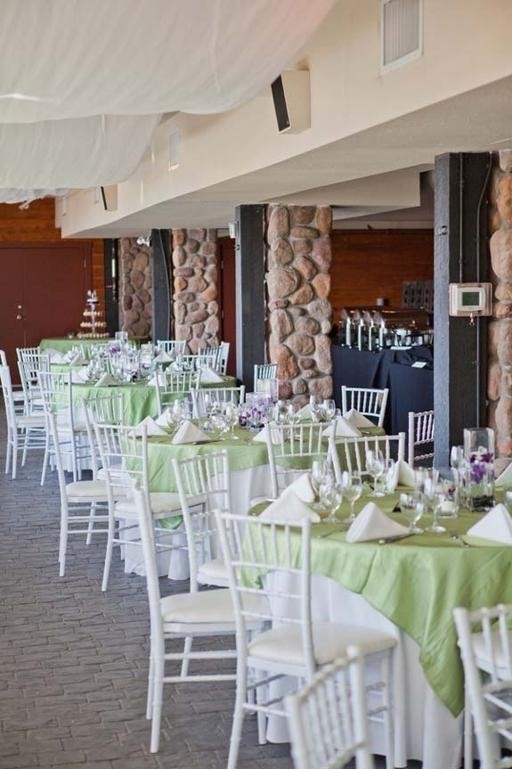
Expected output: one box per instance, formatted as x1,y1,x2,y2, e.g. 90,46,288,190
309,449,461,535
72,331,157,384
175,397,335,439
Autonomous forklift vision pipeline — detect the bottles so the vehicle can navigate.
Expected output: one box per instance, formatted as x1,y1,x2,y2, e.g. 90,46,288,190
339,311,434,355
